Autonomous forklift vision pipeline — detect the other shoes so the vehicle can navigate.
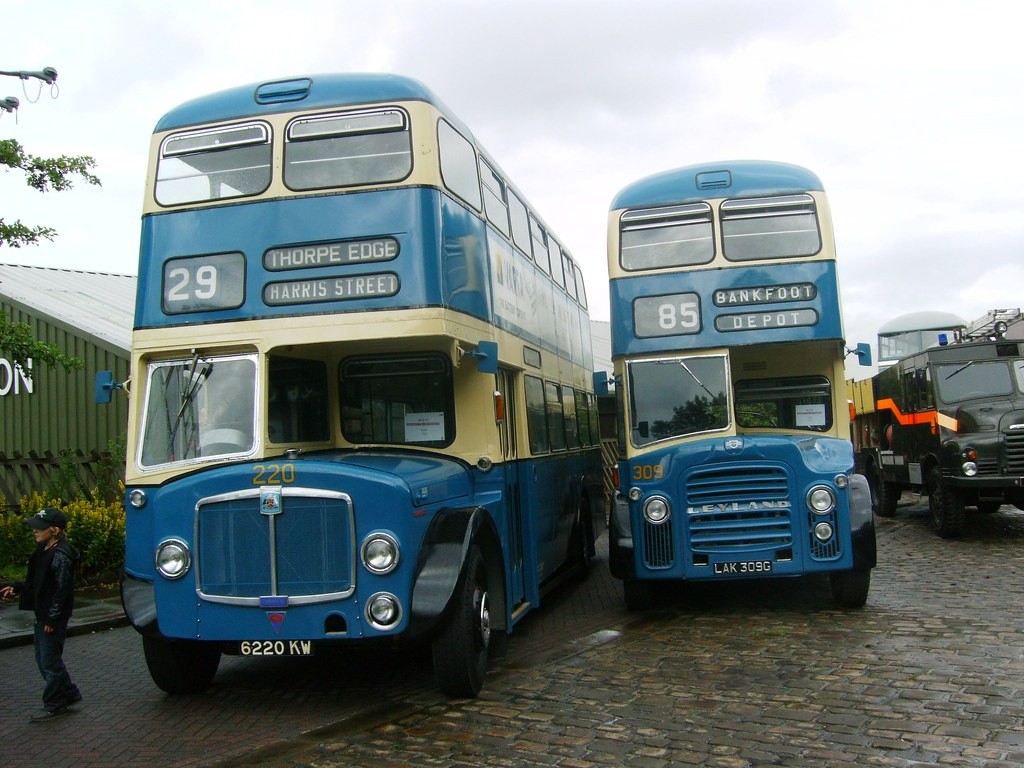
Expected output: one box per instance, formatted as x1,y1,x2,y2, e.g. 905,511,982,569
32,706,69,723
60,691,82,707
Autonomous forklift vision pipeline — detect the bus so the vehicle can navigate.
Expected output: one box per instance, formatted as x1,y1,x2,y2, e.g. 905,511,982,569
93,66,609,701
594,156,877,616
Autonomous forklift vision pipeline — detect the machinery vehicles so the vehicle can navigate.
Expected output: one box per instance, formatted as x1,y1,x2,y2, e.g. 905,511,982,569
847,307,1024,538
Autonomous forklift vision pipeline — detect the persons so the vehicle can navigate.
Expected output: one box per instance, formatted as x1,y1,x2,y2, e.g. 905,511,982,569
0,508,83,720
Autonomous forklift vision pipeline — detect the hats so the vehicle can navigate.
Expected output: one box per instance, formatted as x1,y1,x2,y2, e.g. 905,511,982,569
25,507,68,529
